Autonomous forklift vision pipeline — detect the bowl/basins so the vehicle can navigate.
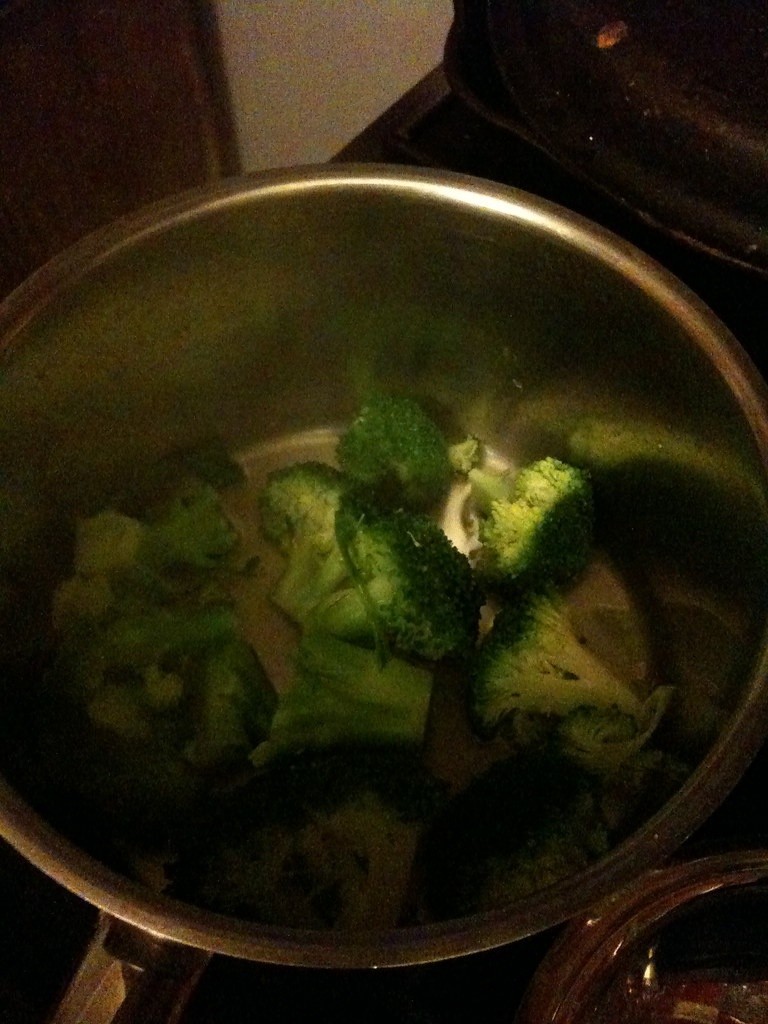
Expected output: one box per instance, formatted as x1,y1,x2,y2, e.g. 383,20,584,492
0,162,767,968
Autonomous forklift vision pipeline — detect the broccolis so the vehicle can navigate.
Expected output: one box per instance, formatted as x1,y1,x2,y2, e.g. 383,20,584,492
48,389,668,936
